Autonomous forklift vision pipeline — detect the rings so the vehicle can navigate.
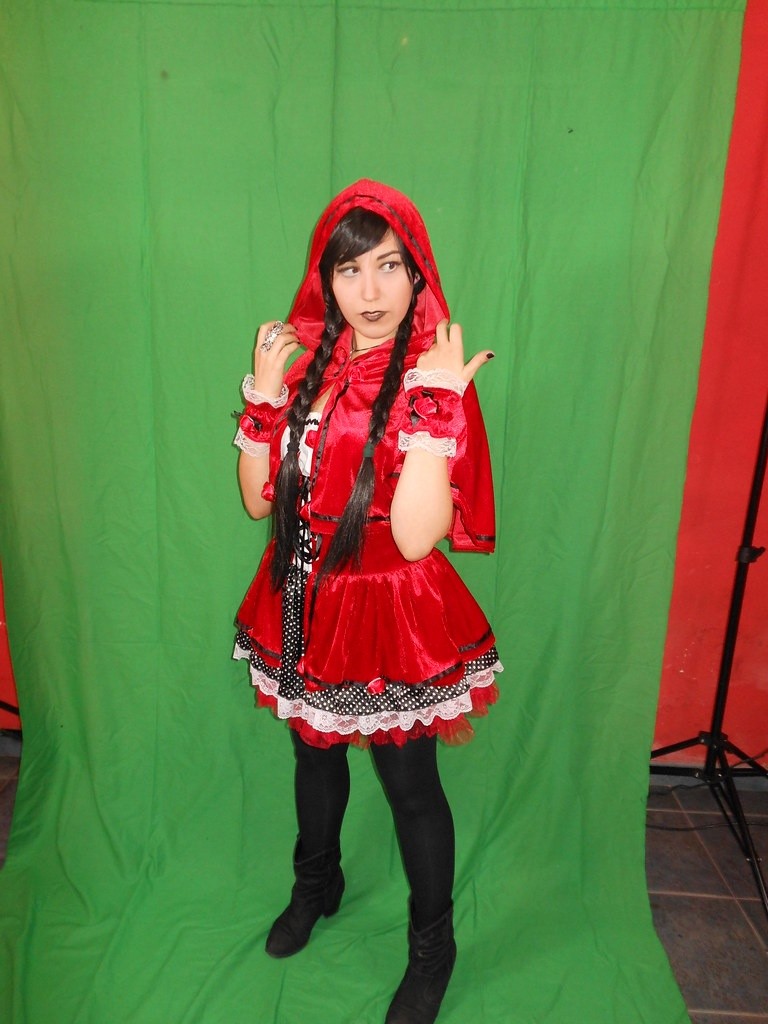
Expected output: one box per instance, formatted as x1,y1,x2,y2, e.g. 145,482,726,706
260,320,284,351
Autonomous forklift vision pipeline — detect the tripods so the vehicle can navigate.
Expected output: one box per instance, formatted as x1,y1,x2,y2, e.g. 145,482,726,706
648,398,767,929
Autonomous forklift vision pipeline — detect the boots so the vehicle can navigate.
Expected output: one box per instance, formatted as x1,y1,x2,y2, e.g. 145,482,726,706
385,897,457,1024
265,833,345,958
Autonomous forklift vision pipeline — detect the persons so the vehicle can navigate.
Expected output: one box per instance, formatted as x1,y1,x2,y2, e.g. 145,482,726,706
228,176,506,1024
